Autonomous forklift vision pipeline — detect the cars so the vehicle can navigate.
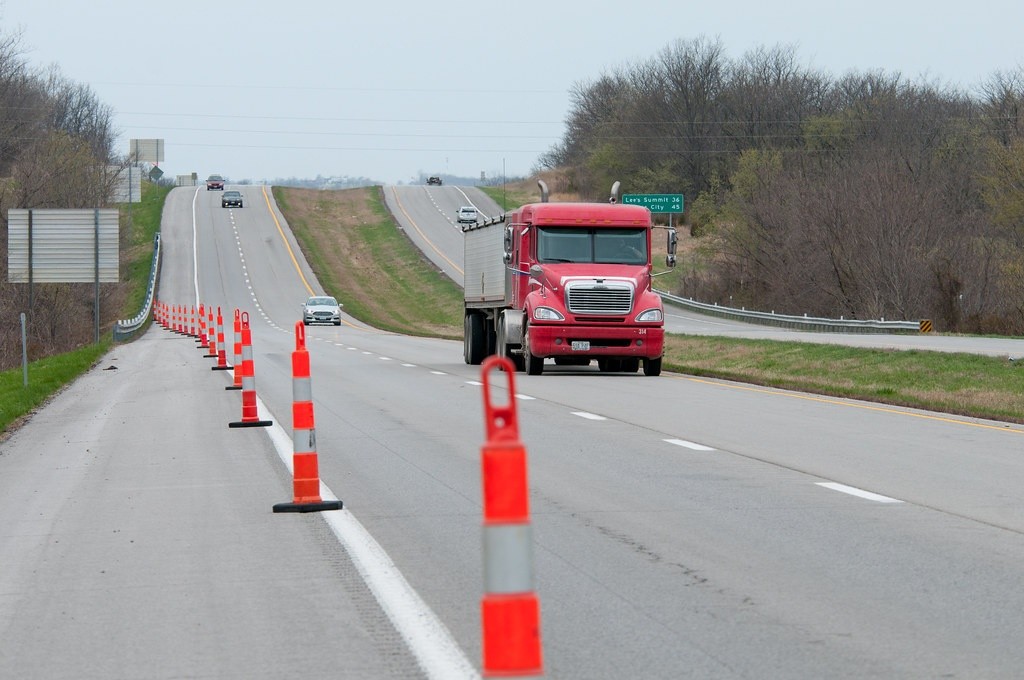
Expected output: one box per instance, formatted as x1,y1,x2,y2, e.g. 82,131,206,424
300,296,345,327
221,189,244,208
206,174,226,191
455,206,478,223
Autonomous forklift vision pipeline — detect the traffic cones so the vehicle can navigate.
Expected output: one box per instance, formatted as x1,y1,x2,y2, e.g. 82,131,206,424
480,355,543,680
152,297,273,428
271,321,344,514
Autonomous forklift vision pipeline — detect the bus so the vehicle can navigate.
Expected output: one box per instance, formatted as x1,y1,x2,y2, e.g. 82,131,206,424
459,177,665,376
